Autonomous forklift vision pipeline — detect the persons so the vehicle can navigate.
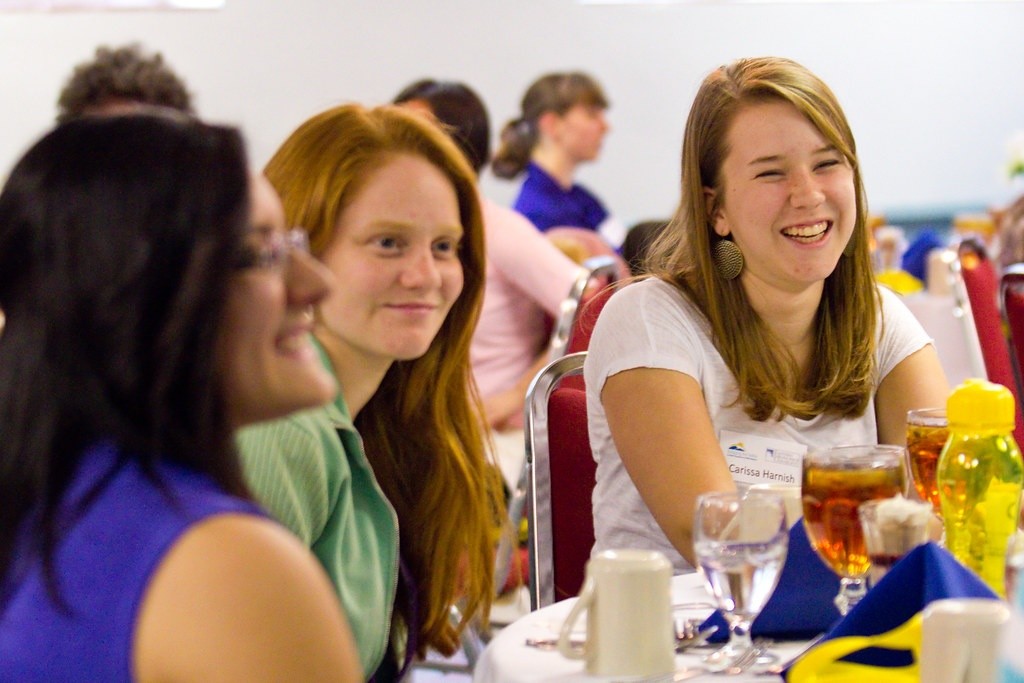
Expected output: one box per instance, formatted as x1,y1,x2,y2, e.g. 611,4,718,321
392,79,601,496
232,109,504,682
56,46,193,120
0,107,368,683
493,73,625,251
583,57,946,577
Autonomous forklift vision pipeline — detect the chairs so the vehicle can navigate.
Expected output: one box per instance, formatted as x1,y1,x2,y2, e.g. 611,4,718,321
494,226,1023,614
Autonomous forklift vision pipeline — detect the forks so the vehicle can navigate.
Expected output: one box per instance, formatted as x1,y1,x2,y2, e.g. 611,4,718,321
676,638,772,680
525,626,718,652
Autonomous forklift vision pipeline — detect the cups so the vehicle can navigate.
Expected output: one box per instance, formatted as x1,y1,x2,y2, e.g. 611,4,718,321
558,549,676,676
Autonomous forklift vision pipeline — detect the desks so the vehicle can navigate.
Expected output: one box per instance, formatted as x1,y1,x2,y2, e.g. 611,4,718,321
470,569,822,683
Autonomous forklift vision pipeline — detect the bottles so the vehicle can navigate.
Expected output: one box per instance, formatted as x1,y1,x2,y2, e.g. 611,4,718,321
858,499,931,592
936,379,1024,602
864,214,889,274
956,215,1002,271
872,226,926,298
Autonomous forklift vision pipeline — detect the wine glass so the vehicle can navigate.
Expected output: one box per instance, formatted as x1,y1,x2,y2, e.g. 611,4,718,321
906,408,975,550
802,446,910,614
693,492,778,665
